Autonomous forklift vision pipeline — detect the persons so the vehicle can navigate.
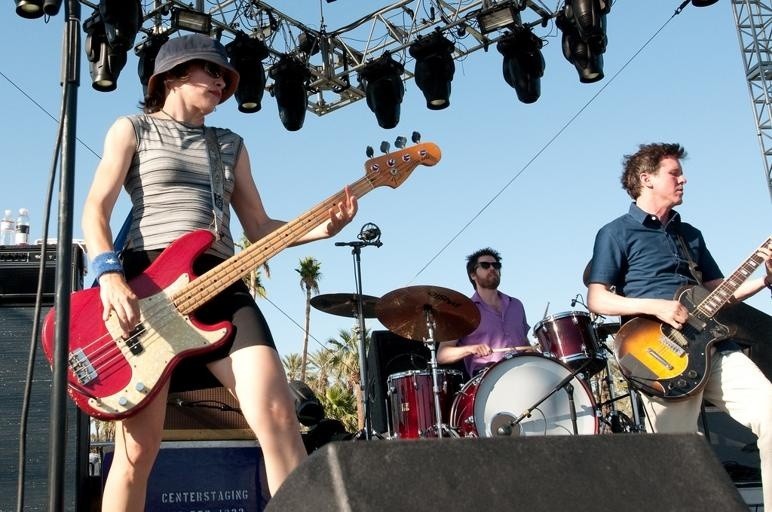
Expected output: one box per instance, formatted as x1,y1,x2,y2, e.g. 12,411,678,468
82,34,309,512
586,135,772,512
437,246,529,379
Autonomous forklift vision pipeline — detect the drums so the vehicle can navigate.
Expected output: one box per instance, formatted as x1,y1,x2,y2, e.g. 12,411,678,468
533,311,621,374
388,370,464,439
451,353,600,436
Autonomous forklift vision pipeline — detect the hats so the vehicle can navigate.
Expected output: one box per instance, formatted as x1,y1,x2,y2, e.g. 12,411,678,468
147,34,240,104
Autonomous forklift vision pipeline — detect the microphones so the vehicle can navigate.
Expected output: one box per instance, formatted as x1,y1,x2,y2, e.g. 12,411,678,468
571,294,606,320
357,222,382,245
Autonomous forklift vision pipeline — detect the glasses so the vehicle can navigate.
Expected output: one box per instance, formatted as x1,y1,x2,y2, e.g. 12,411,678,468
474,261,502,269
197,61,222,79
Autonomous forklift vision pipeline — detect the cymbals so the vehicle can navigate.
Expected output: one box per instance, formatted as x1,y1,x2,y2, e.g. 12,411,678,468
309,293,379,318
377,285,480,340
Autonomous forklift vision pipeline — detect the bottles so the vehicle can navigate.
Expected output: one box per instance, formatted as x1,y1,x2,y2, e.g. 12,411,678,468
16,208,30,245
0,209,16,245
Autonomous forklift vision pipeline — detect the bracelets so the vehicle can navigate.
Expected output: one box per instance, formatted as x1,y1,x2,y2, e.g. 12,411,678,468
764,277,771,287
89,252,125,281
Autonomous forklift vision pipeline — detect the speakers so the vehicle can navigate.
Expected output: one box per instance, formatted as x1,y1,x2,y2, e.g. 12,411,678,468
1,291,92,511
367,330,430,435
697,301,772,486
269,432,751,512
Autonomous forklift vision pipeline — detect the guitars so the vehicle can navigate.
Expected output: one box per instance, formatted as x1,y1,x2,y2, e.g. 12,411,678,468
42,133,441,419
614,236,772,402
